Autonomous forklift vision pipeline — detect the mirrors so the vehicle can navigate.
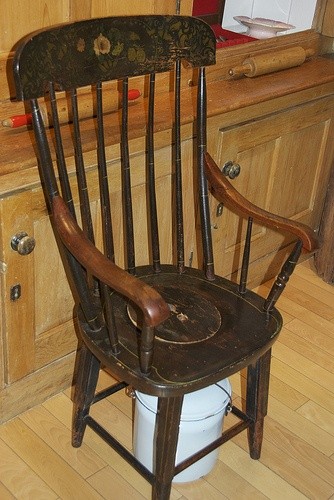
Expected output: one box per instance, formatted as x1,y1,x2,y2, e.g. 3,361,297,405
178,0,330,61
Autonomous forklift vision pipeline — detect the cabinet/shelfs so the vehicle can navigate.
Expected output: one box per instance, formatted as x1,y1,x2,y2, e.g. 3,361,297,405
208,81,334,310
1,129,208,430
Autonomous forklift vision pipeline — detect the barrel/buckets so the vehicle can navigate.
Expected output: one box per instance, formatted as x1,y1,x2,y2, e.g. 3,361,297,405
133,376,231,482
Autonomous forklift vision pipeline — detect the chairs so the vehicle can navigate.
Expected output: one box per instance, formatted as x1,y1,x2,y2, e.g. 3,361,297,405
6,14,321,500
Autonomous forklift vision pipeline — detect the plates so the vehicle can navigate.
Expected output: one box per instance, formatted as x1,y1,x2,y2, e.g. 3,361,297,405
233,16,295,40
